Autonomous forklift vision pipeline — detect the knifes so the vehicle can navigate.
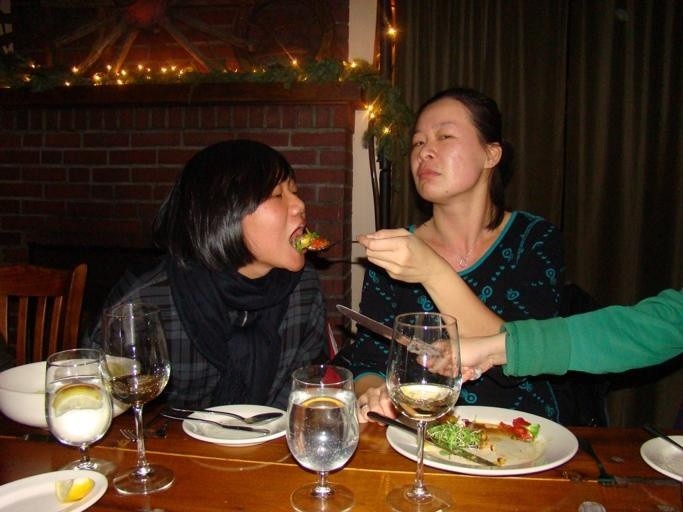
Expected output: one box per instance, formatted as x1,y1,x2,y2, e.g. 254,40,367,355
157,413,280,436
335,304,482,381
368,411,500,468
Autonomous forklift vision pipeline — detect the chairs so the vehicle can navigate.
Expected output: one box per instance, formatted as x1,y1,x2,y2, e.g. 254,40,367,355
0,259,89,369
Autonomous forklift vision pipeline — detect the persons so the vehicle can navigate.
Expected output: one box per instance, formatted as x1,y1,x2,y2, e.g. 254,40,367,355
90,140,331,418
349,89,578,429
417,289,682,383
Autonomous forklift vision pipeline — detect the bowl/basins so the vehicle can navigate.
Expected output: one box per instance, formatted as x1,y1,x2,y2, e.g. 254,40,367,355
0,355,141,432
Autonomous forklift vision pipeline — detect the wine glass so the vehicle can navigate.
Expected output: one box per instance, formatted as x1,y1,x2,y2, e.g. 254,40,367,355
385,311,462,512
285,364,359,512
44,302,175,496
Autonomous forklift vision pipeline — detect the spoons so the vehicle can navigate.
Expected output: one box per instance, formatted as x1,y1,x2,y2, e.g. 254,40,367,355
301,237,360,252
168,407,282,426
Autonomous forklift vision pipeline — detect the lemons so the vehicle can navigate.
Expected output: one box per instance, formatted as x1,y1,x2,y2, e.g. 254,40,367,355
55,477,95,502
52,385,102,417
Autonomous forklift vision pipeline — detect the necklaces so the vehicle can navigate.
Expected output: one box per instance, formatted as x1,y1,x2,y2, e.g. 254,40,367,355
457,245,474,267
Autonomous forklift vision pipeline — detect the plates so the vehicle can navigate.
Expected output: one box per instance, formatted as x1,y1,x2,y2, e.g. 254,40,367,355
0,469,109,512
640,435,683,483
182,403,287,448
386,405,581,477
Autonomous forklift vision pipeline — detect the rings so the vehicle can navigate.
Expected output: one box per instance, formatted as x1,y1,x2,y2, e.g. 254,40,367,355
360,404,369,409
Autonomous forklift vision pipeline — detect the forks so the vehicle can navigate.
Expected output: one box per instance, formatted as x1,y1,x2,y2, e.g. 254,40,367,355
579,436,618,493
119,412,164,441
